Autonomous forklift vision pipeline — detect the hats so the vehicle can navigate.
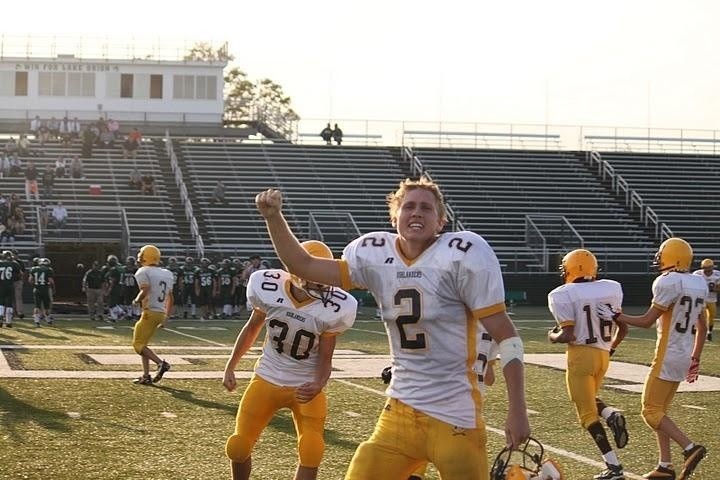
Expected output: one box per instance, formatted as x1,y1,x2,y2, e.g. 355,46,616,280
11,249,20,255
93,260,100,266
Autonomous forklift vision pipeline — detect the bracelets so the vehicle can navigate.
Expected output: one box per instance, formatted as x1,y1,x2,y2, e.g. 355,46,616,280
612,312,622,321
691,355,700,363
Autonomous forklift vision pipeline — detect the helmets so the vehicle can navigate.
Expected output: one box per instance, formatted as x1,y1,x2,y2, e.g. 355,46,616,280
650,238,693,274
558,249,598,284
2,251,14,261
101,255,136,275
260,259,272,269
701,259,714,270
286,240,334,294
159,256,251,271
32,256,52,268
490,453,568,480
135,244,161,266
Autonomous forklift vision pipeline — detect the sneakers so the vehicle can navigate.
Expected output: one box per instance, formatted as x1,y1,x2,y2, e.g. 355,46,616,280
643,463,676,479
606,411,629,449
152,359,170,383
0,311,53,329
169,312,252,321
592,465,625,478
679,445,708,479
706,330,712,343
132,374,153,386
89,309,142,324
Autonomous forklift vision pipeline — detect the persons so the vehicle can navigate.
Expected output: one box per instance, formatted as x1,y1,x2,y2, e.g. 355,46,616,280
130,244,176,386
596,237,710,480
1,113,159,243
320,122,333,145
545,249,628,479
254,175,564,479
0,248,272,326
222,240,358,478
208,180,230,207
692,259,719,340
333,123,344,145
